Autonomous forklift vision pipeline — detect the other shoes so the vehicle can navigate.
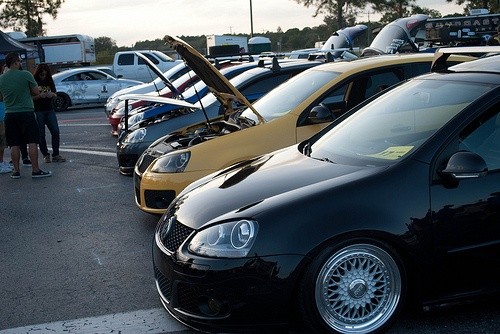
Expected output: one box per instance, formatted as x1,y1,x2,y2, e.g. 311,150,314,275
43,155,51,163
0,162,12,173
52,155,66,162
22,159,32,167
31,169,52,177
9,171,20,178
8,161,14,167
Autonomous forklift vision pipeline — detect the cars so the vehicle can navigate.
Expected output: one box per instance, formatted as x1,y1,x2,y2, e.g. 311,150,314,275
51,68,147,112
151,55,500,334
101,14,443,176
132,34,484,216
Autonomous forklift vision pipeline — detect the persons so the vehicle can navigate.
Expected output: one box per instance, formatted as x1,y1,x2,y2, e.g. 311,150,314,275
30,62,66,162
0,52,52,179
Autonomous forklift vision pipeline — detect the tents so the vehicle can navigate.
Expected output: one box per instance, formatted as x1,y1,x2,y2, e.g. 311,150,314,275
0,30,38,64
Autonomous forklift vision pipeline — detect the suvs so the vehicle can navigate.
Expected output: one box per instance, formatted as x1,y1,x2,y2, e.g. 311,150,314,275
114,50,186,85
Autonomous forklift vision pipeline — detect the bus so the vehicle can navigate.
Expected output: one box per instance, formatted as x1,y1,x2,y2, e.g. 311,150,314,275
0,31,96,66
